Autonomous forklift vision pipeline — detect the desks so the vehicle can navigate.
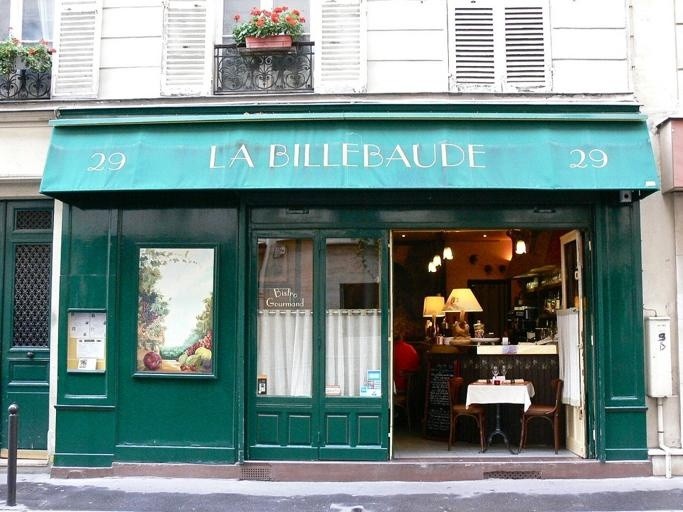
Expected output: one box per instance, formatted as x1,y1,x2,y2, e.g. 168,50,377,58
466,380,534,454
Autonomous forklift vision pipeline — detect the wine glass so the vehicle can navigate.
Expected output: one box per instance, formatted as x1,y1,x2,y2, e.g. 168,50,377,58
491,366,499,379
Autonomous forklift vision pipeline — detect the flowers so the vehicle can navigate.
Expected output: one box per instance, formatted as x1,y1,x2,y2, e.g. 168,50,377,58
0,26,21,71
233,6,307,41
22,40,56,74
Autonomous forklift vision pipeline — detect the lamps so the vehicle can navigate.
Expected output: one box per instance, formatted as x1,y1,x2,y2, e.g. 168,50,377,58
443,288,483,321
423,296,446,339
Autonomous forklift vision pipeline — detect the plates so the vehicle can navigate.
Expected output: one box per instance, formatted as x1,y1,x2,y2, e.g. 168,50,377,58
470,337,500,342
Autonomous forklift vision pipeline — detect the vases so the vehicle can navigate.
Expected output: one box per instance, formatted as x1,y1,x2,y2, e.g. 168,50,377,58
246,36,291,49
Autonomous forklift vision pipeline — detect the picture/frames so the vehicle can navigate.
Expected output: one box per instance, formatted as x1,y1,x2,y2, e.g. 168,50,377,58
132,241,219,379
422,351,459,443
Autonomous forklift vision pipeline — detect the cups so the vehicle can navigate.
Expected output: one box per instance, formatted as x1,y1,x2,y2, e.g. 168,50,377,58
486,379,490,384
500,364,508,375
543,270,561,284
511,379,515,384
436,336,443,345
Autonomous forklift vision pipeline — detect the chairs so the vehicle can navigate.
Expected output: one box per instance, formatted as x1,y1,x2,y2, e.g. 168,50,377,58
447,378,485,452
518,380,561,454
393,370,419,434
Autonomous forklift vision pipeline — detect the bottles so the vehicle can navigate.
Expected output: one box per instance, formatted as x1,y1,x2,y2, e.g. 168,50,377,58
473,320,484,338
513,304,527,310
535,319,555,341
542,289,560,314
